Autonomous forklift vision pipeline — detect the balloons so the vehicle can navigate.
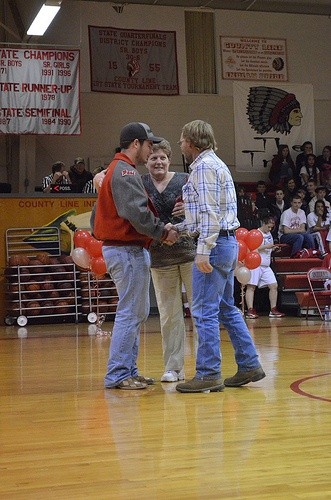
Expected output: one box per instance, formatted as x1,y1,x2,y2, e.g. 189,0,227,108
72,230,107,274
234,228,264,284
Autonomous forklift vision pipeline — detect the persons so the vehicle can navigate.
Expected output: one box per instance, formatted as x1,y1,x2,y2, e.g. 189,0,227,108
42,159,105,194
233,141,331,258
163,120,267,393
93,139,197,382
89,122,183,390
242,216,285,319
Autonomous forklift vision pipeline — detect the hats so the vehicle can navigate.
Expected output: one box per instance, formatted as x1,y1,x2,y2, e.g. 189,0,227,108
120,122,162,144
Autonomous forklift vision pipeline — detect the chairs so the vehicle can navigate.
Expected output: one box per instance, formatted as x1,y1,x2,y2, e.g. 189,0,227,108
306,268,331,320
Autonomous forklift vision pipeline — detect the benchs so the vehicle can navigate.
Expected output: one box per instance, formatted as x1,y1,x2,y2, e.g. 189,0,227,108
238,181,331,310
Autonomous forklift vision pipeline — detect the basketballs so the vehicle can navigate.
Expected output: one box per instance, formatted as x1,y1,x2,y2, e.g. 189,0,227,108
8,252,120,316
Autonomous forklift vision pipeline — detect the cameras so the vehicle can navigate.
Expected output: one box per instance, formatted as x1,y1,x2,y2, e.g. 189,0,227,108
273,247,281,252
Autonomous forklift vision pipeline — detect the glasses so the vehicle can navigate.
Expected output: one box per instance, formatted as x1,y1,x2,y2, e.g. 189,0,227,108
176,139,186,146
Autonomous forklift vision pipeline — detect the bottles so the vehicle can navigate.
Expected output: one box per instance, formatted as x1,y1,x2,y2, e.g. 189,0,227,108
324,306,329,322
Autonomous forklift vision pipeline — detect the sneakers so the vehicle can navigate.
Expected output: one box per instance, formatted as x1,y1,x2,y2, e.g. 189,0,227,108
269,307,285,317
115,377,147,389
161,370,184,382
246,308,259,319
134,375,155,384
224,367,266,386
176,376,225,392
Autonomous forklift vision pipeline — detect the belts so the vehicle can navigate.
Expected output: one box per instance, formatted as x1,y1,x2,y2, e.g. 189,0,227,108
220,229,235,236
